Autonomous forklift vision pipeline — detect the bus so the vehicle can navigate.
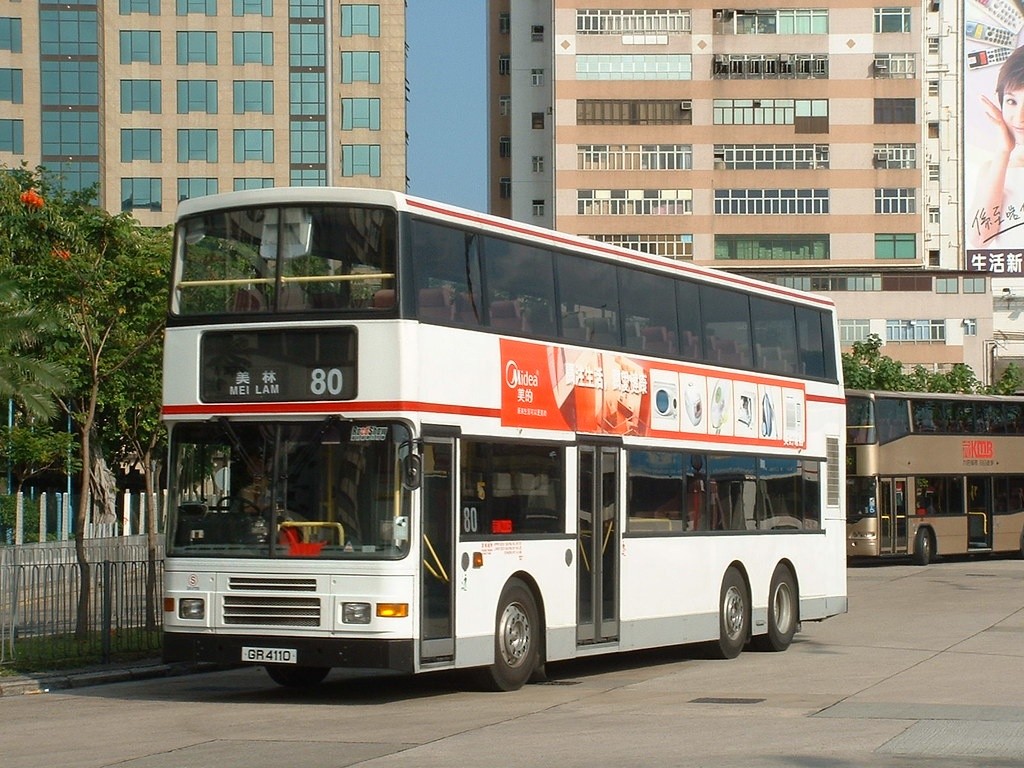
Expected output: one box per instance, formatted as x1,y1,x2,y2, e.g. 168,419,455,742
845,388,1024,566
161,187,850,693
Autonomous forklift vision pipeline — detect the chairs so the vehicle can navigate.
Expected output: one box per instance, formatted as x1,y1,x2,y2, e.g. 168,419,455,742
847,416,1024,445
231,276,809,375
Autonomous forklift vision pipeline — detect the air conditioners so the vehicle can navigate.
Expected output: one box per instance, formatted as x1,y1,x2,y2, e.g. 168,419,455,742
876,58,932,244
785,393,803,431
547,104,552,116
680,101,692,110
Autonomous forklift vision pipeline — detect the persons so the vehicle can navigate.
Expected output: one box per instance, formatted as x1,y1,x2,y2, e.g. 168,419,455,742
967,43,1024,250
